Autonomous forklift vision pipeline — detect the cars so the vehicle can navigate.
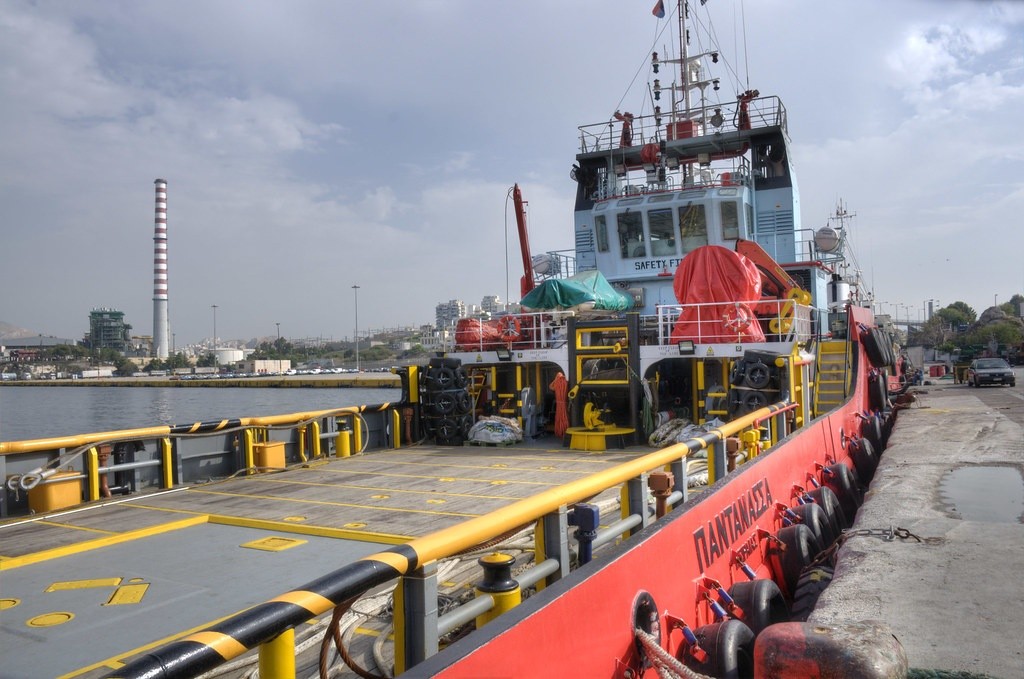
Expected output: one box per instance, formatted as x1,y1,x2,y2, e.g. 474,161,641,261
967,357,1016,388
168,366,402,379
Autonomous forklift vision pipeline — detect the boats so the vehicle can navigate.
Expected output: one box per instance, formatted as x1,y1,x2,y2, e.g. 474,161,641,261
1,1,907,679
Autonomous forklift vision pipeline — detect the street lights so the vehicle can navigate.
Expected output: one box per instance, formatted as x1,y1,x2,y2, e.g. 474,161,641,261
352,284,360,372
276,322,281,376
211,304,220,376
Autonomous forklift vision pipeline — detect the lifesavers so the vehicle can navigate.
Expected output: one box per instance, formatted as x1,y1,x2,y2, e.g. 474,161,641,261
718,359,771,422
497,315,521,342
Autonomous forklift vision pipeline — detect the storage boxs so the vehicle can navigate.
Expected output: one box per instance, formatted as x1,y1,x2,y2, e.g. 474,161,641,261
26,464,82,515
252,441,286,473
